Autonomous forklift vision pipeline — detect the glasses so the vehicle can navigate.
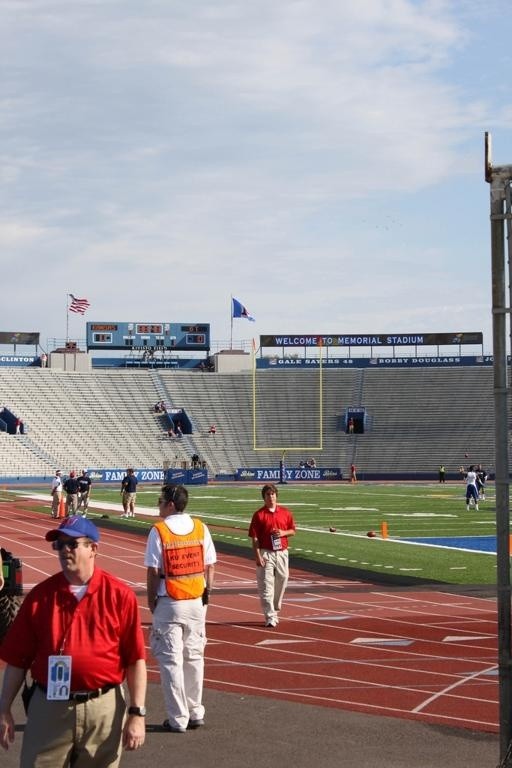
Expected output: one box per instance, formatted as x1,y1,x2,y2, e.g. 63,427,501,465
171,484,179,499
51,539,91,551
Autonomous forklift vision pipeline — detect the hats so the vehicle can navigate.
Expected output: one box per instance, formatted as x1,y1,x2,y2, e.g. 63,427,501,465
45,518,100,539
55,468,87,478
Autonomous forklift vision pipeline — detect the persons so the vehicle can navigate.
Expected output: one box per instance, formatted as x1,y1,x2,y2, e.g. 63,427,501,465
51,469,91,519
155,401,166,413
198,359,206,371
142,483,218,733
0,514,148,767
207,360,215,372
168,425,182,437
16,415,22,434
459,464,487,512
299,457,316,469
192,454,206,469
351,464,357,483
349,417,354,434
120,469,138,518
40,353,49,368
0,551,7,591
210,424,216,434
248,484,296,626
440,465,445,483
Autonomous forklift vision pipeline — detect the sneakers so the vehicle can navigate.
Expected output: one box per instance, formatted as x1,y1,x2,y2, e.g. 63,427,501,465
265,617,277,628
162,716,188,732
120,513,134,518
189,719,204,726
51,512,89,520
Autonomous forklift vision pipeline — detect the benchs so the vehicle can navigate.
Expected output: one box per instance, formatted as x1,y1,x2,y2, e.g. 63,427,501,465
0,364,512,488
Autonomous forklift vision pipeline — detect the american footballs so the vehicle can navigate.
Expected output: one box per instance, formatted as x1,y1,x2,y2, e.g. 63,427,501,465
330,527,337,531
367,530,377,536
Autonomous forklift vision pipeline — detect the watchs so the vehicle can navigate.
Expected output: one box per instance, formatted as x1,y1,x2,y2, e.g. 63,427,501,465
128,705,149,716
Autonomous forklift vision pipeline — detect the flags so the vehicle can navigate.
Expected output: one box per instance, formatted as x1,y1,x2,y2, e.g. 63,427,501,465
68,290,91,316
231,296,255,322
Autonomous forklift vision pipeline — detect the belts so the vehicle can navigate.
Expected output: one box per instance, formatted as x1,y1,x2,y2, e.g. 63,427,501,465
34,681,116,703
